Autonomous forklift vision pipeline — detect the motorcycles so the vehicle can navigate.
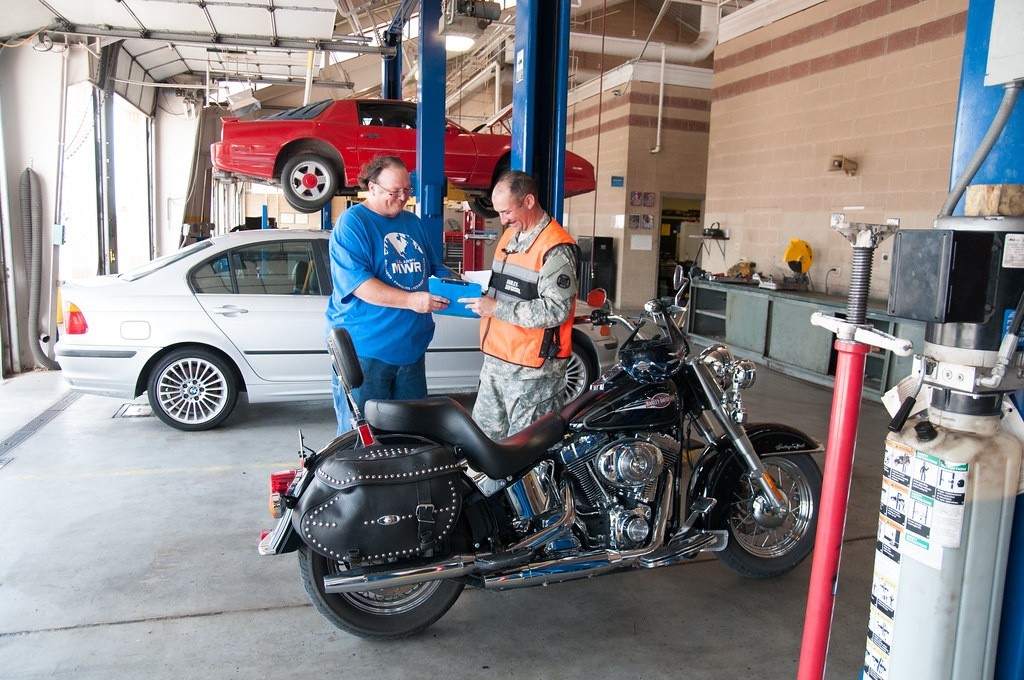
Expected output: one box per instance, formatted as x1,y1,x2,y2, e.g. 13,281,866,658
255,259,825,644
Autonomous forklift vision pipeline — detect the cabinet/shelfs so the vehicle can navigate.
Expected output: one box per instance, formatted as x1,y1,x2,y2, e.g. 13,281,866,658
684,279,929,405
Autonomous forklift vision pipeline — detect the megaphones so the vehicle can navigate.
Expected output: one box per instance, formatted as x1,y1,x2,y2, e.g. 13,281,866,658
826,153,858,173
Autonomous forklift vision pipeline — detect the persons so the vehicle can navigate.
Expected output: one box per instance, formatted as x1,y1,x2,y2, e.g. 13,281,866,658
457,170,578,442
324,157,454,438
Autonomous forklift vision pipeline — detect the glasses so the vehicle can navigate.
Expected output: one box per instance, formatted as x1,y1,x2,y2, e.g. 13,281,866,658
376,182,414,198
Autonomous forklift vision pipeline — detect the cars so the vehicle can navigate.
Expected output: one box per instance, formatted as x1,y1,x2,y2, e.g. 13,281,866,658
207,96,598,220
49,224,618,431
